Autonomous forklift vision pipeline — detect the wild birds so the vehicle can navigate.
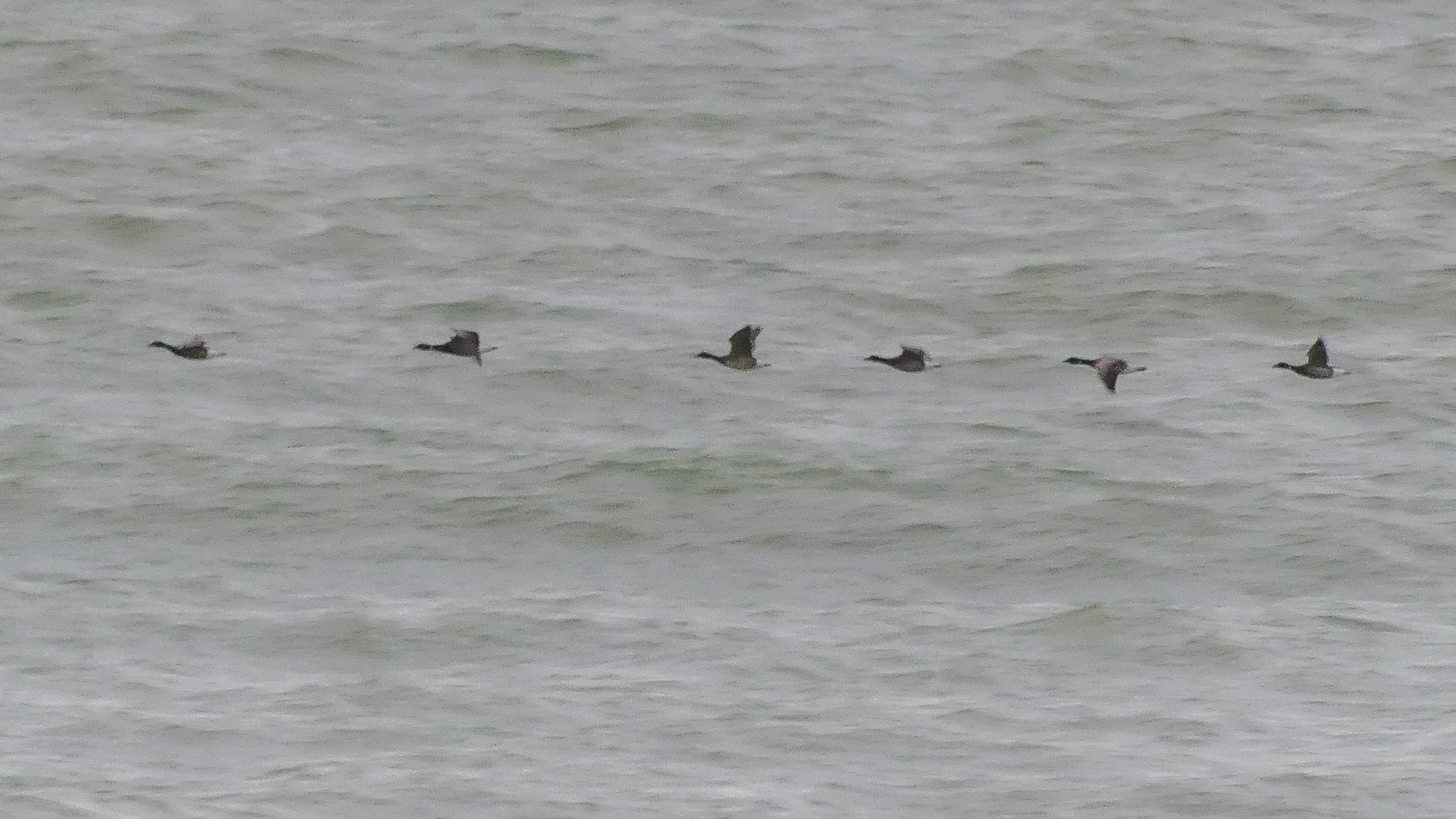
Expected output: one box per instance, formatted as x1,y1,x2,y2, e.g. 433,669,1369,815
1272,335,1353,382
147,337,229,362
864,345,943,374
690,324,772,372
1061,356,1149,397
412,325,499,367
